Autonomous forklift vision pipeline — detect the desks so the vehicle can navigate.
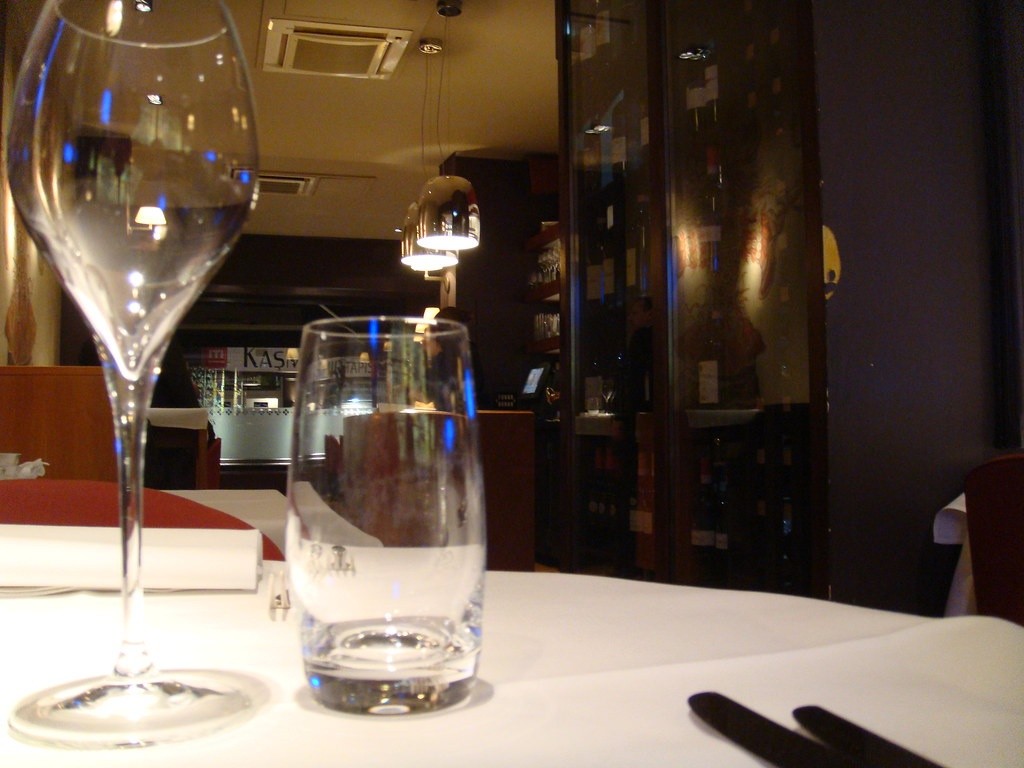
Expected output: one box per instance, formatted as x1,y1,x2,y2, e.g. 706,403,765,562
575,414,614,548
635,409,791,588
163,490,288,557
0,561,1024,768
146,407,208,490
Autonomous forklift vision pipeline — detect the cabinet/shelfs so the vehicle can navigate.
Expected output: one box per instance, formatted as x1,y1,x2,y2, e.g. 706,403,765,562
523,222,560,355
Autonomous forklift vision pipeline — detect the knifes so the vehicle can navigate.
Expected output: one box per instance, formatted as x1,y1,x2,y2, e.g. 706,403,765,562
791,704,942,768
687,691,862,768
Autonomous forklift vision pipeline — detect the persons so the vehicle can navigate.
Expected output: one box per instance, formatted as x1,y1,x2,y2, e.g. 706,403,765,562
611,298,654,575
425,307,486,411
80,333,215,489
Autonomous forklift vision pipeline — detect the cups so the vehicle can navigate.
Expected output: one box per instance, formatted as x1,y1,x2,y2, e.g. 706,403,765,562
283,314,489,718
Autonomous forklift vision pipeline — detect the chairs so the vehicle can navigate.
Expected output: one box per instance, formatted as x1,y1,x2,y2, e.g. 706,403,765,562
965,452,1024,626
0,477,285,562
743,403,811,591
0,365,117,482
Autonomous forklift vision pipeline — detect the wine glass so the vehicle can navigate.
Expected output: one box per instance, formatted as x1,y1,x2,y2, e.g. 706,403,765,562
1,0,269,751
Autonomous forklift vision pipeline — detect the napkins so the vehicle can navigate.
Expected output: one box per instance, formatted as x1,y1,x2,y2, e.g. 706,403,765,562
0,453,45,480
0,522,263,591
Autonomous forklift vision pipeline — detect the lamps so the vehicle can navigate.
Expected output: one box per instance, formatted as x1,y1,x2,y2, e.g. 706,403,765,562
415,0,481,251
400,37,460,271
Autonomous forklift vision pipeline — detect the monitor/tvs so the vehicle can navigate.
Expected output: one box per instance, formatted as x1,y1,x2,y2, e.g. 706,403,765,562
522,363,551,399
